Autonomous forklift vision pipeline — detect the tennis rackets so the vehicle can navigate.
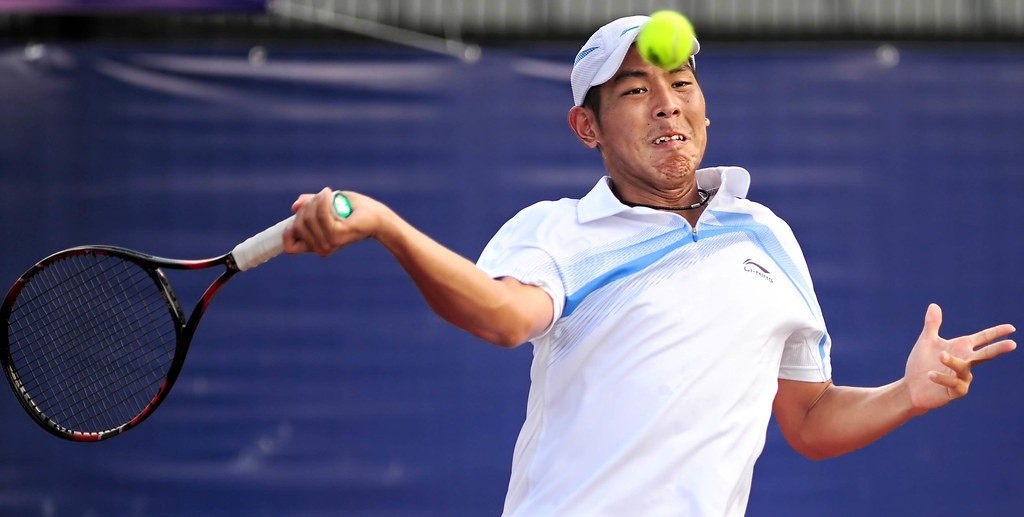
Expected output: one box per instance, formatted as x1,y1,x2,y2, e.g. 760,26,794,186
1,189,353,441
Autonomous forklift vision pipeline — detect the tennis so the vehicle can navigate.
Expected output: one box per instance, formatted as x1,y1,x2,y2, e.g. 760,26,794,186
637,10,696,70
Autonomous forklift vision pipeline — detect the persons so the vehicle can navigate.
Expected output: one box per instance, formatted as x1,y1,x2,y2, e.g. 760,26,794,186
282,16,1017,517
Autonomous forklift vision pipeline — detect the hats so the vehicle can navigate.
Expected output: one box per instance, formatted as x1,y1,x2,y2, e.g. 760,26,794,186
571,10,700,107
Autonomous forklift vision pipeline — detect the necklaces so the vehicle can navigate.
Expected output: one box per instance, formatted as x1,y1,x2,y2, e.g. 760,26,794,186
623,189,709,212
